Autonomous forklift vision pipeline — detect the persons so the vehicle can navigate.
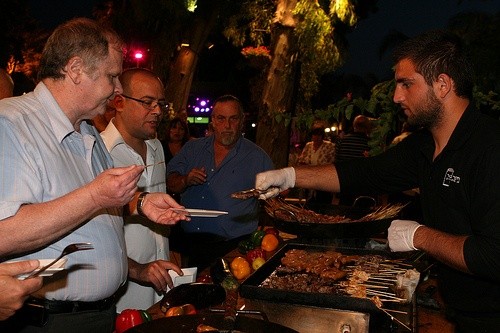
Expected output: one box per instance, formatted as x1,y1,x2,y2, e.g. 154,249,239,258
289,112,412,204
162,116,213,163
255,38,500,333
0,259,46,322
102,68,184,315
0,70,115,133
166,94,277,272
0,18,191,332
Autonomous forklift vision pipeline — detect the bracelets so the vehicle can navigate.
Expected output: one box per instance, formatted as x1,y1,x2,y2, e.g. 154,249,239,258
136,192,148,216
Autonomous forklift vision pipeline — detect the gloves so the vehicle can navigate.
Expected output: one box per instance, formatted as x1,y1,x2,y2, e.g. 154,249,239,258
388,220,425,252
256,166,296,199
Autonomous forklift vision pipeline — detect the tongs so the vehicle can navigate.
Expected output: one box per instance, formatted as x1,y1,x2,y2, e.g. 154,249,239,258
231,186,282,201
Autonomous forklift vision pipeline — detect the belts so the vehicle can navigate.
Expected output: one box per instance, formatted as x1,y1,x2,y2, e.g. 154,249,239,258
24,280,130,314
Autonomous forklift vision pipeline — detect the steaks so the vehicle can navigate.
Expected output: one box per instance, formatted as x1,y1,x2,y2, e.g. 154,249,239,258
231,190,259,200
282,249,362,280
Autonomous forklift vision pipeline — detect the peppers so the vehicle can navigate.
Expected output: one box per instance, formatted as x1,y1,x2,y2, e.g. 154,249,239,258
165,304,197,317
221,227,279,289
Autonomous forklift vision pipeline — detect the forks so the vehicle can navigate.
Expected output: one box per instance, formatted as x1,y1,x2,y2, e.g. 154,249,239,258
23,242,95,280
145,161,164,168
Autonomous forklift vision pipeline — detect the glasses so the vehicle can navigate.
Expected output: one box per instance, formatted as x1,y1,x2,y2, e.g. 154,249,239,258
124,95,169,113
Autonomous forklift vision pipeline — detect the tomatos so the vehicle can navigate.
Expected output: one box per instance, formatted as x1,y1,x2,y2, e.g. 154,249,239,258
114,309,143,333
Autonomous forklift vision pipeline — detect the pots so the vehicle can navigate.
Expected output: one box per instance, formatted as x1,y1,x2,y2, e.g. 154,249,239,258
261,196,403,238
122,310,300,333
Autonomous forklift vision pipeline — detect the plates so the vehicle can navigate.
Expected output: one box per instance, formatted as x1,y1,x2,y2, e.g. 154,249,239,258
166,267,197,293
7,259,65,278
169,207,228,217
